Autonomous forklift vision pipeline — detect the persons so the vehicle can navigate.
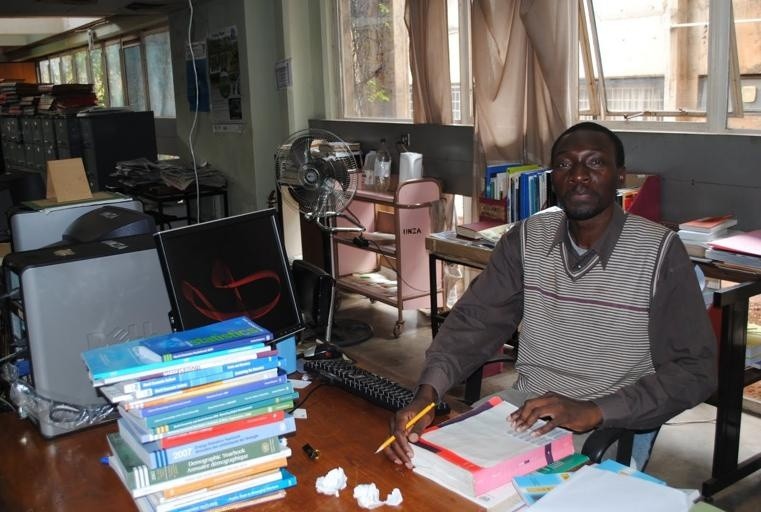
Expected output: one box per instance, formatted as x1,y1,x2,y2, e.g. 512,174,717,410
383,122,720,470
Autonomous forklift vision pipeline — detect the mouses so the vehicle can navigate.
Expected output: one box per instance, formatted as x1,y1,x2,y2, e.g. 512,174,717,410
303,344,342,360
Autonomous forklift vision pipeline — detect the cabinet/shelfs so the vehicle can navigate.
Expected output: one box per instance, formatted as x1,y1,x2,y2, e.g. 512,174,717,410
0,108,158,197
329,170,443,336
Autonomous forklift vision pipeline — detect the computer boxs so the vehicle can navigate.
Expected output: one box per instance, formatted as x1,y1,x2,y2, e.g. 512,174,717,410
1,243,174,440
7,192,145,254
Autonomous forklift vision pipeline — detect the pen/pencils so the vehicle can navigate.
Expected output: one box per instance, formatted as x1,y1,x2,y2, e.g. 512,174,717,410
374,402,436,455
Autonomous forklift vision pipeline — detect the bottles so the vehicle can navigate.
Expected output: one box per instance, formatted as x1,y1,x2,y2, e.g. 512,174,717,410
365,138,391,192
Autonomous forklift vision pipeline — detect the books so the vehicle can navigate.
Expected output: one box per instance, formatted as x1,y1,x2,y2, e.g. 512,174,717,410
0,78,99,117
81,318,297,511
615,187,639,212
274,140,360,188
402,395,723,511
456,218,512,247
677,214,761,273
485,165,553,225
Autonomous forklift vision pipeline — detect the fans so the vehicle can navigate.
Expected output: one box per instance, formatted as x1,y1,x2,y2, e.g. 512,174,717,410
272,125,374,348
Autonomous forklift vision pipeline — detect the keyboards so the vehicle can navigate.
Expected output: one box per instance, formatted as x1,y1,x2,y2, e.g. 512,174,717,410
304,358,451,416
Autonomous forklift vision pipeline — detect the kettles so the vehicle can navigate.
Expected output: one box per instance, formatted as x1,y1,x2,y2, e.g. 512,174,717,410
399,151,423,186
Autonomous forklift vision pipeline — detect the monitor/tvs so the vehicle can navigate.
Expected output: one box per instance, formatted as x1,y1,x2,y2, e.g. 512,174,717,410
153,207,307,346
61,205,156,243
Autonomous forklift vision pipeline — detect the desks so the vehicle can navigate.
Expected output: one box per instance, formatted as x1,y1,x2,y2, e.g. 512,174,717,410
424,221,761,497
104,180,229,233
0,330,586,511
